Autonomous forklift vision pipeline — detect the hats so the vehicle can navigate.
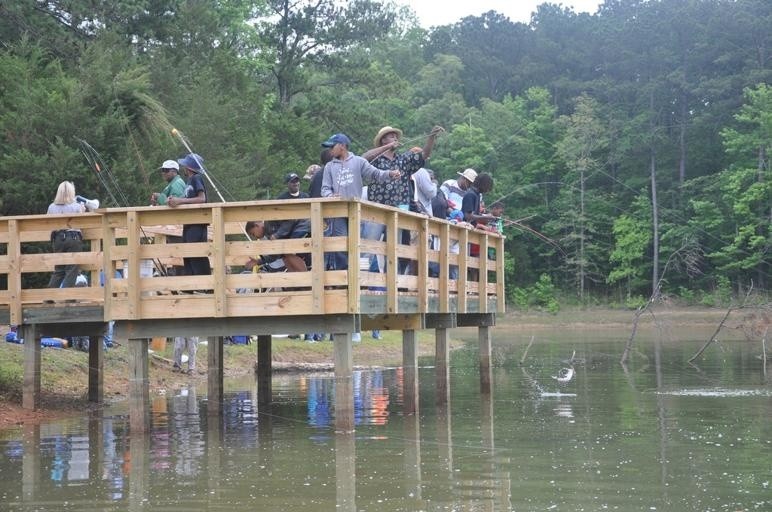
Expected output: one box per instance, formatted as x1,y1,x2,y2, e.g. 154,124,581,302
303,164,322,180
178,154,208,176
321,134,351,148
456,168,478,183
374,126,403,149
285,173,302,184
158,160,180,172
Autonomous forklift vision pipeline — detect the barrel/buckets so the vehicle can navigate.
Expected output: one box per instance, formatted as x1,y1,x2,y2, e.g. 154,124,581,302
123,259,152,296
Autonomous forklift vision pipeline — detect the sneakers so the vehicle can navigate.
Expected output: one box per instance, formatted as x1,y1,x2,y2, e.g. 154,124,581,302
173,366,195,375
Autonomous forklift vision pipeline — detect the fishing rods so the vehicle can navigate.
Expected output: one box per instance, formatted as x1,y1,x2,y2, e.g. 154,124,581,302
485,182,565,211
369,131,435,164
483,214,567,257
172,128,267,270
327,155,344,270
419,127,523,210
72,118,177,294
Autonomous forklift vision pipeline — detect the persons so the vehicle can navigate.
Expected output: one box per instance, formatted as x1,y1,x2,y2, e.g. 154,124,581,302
245,124,501,293
43,180,100,305
287,328,381,347
221,265,249,347
61,254,123,349
171,335,200,375
151,154,215,294
